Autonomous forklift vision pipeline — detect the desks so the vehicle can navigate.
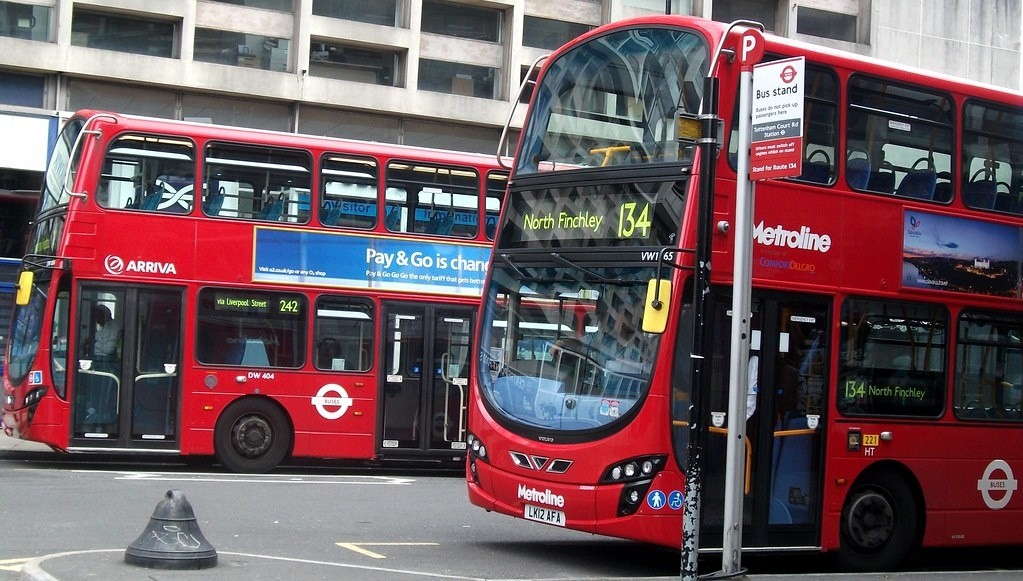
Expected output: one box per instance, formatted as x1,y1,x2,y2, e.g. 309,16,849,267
435,76,474,96
309,59,382,84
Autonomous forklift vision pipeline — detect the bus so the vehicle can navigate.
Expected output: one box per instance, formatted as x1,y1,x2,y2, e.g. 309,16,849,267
461,13,1023,572
0,107,611,476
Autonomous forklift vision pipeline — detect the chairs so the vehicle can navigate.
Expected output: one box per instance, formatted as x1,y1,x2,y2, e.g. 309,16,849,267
965,400,1023,419
123,181,495,241
316,338,340,369
798,145,1023,213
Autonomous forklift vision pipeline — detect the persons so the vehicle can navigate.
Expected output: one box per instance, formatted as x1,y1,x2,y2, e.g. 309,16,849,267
317,338,353,371
88,305,123,357
551,289,657,396
